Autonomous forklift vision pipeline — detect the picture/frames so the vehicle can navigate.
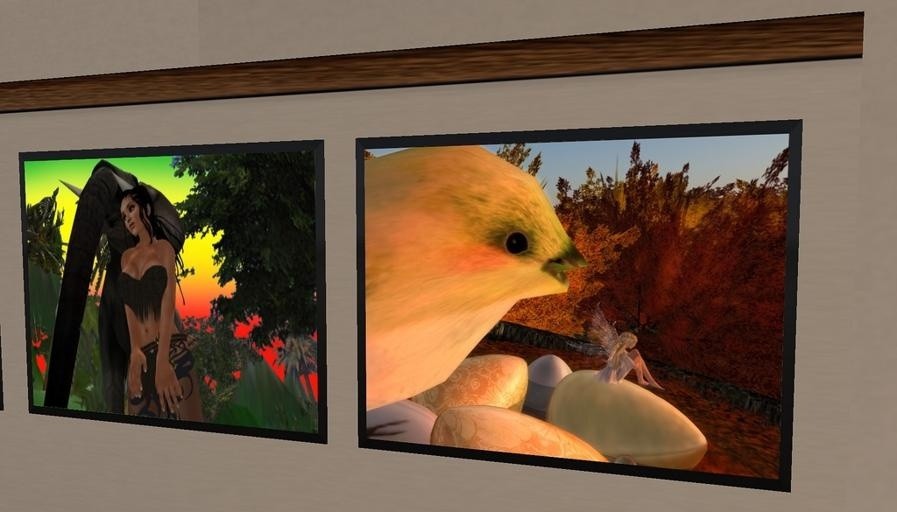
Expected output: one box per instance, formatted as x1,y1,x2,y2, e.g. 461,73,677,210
19,139,329,444
355,117,804,494
